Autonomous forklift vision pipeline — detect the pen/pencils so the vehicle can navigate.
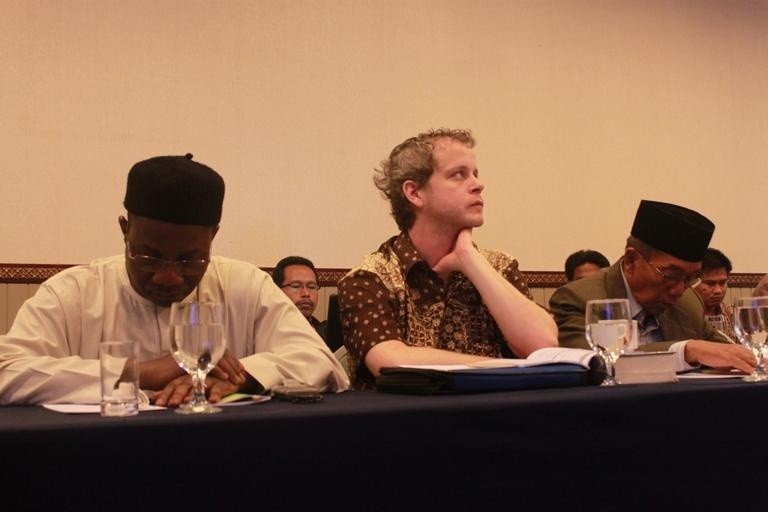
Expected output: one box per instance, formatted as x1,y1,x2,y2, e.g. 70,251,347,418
717,330,767,375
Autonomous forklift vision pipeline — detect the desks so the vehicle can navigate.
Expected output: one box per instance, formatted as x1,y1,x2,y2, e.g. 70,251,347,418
0,355,768,512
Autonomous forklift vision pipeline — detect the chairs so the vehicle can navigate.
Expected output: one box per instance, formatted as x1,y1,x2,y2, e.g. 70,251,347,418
327,291,342,352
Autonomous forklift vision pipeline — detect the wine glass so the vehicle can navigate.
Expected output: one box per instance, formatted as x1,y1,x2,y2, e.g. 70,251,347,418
166,299,227,415
583,296,633,387
730,294,768,383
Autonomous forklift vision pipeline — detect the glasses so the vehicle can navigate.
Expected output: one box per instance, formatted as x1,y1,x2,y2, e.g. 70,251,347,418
653,267,703,288
277,281,321,291
124,227,214,274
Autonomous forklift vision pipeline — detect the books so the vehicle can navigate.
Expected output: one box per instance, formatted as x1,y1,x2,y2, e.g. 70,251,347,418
612,350,677,387
373,344,609,396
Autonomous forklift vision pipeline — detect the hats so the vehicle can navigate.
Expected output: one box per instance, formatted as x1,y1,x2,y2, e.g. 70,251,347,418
124,154,227,228
632,198,715,265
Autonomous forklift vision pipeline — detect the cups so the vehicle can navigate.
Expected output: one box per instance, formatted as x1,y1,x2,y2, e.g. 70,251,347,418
97,340,140,417
706,313,728,334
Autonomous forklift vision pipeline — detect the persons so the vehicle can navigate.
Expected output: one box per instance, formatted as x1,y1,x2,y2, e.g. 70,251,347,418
270,254,326,338
0,150,356,413
564,249,611,281
546,196,758,376
689,246,746,344
335,127,560,382
